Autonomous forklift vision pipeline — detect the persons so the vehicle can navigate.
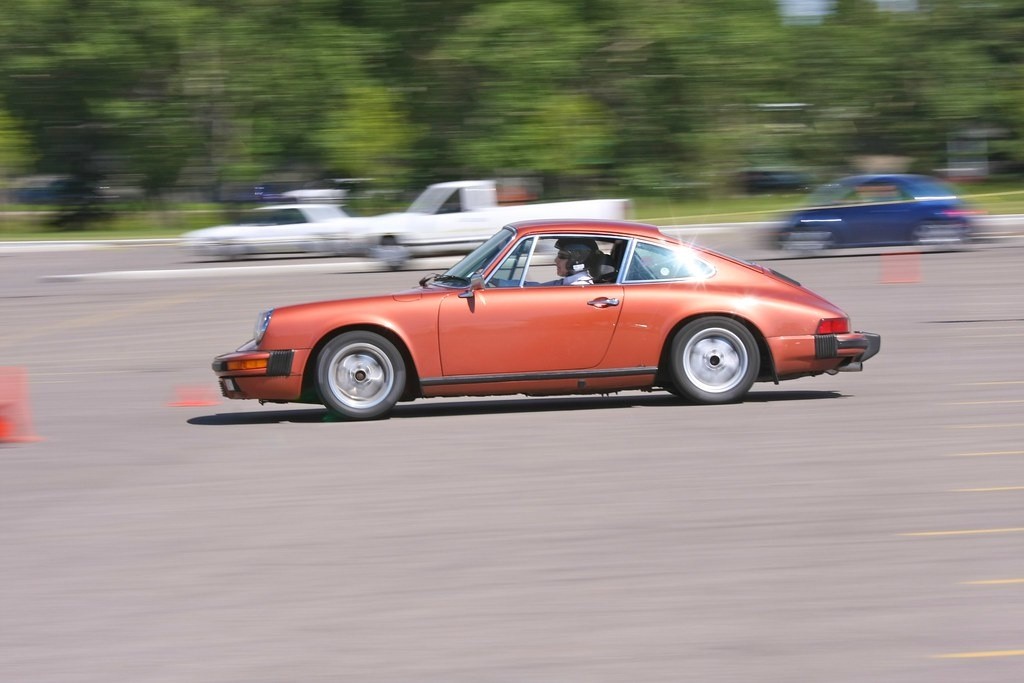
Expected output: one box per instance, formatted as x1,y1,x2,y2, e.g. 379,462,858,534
474,237,600,288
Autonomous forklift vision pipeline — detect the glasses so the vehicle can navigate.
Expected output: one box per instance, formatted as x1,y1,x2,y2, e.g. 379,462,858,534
558,252,570,259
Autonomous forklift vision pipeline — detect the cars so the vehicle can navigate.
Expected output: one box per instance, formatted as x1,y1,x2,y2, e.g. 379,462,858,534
211,220,881,425
171,203,378,260
766,170,983,250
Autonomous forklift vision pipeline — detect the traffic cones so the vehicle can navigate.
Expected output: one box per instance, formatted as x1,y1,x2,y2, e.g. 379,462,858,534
0,364,41,447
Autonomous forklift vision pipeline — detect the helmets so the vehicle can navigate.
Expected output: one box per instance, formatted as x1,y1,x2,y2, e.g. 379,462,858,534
555,238,598,267
589,254,619,284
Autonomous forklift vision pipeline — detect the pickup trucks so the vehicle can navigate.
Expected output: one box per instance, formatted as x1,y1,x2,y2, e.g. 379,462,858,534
335,178,635,273
